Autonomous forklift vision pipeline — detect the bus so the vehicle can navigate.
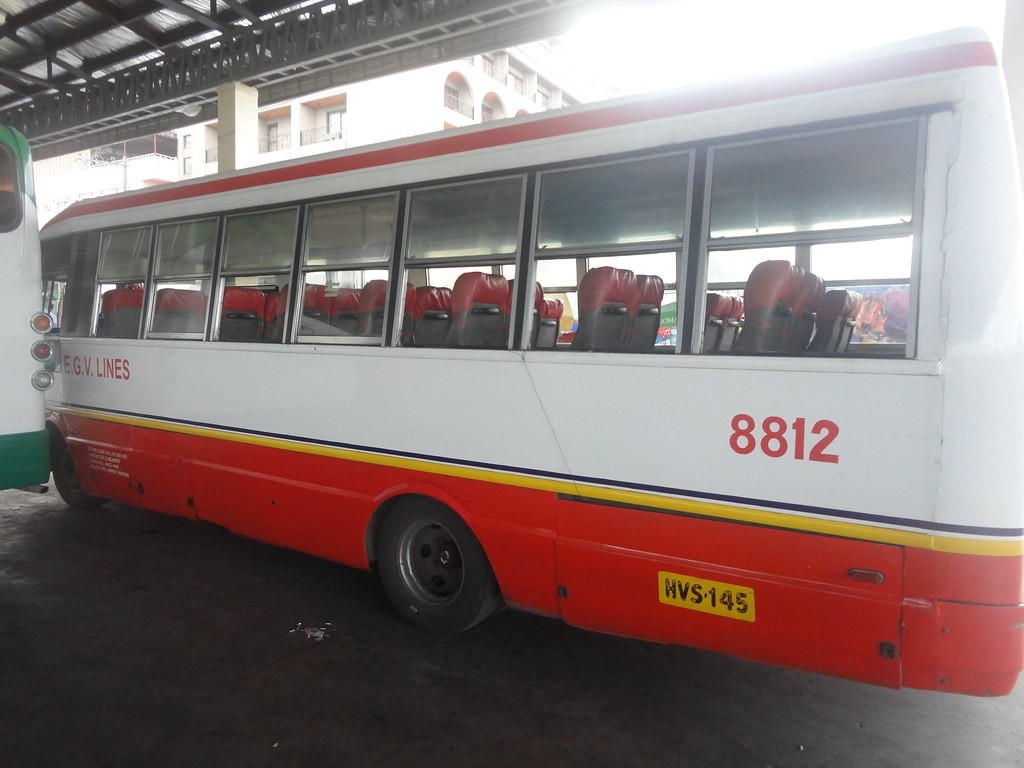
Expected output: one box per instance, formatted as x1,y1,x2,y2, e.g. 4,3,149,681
37,28,1022,701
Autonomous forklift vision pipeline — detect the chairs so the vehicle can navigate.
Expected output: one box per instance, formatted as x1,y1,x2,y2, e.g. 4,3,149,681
95,260,865,356
